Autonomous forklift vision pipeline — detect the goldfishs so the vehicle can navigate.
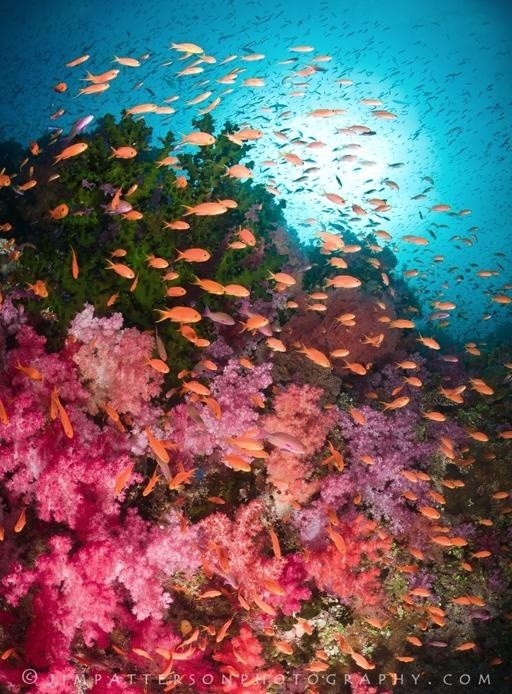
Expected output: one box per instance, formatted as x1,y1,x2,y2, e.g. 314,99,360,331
0,0,512,694
